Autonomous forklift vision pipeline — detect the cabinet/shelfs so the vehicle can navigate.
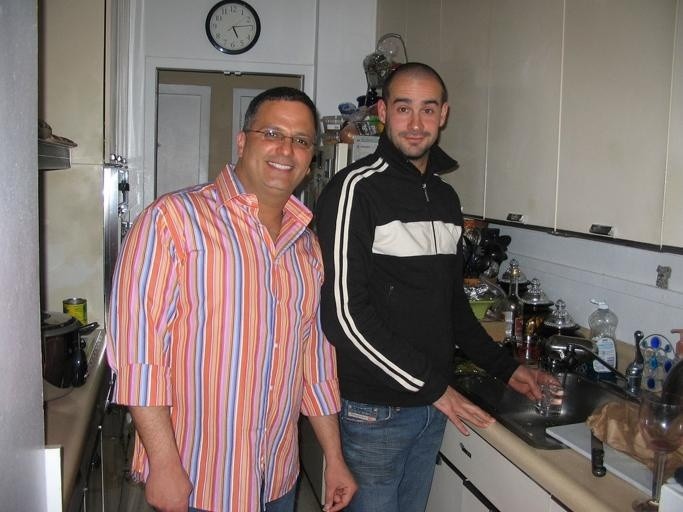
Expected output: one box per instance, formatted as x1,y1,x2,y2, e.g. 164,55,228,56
428,418,567,512
375,0,481,216
558,2,682,254
482,0,556,236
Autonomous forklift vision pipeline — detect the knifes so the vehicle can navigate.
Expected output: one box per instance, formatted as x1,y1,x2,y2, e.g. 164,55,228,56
590,429,606,476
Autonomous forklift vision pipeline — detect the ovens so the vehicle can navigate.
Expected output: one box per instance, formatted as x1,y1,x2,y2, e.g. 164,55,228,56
104,164,133,307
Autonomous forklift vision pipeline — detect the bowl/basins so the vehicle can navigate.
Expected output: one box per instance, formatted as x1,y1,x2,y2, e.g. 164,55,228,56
465,293,494,320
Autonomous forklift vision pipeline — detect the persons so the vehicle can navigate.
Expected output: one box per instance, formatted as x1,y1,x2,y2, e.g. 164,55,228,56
106,87,358,512
315,62,564,512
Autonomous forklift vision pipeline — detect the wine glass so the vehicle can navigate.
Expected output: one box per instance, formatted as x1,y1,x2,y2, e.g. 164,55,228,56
631,391,681,512
536,356,562,419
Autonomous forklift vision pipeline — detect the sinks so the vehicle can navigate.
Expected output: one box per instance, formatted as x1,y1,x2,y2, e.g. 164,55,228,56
453,369,643,450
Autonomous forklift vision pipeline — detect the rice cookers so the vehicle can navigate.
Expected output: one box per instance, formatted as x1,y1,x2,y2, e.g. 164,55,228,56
40,311,83,404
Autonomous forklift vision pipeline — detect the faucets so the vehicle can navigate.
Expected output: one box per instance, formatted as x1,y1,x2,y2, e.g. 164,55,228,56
566,330,647,394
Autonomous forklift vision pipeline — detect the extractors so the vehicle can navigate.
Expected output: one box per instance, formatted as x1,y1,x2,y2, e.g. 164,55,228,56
37,139,70,170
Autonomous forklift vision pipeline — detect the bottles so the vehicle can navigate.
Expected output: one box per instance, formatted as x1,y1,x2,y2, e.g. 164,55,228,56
497,270,578,370
500,259,527,299
588,298,618,379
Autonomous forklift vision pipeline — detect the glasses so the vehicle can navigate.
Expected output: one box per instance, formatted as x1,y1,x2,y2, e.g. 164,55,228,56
243,127,317,149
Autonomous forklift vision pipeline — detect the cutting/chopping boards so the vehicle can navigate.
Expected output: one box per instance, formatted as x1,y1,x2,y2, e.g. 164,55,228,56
546,422,661,498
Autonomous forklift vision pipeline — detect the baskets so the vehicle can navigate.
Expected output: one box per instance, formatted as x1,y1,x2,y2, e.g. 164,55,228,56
469,298,500,320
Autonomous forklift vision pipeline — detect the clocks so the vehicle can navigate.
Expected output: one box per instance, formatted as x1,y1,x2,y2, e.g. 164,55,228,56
205,1,263,56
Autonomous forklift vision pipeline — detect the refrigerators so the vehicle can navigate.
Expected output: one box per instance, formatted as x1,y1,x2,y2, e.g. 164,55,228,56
305,132,381,231
